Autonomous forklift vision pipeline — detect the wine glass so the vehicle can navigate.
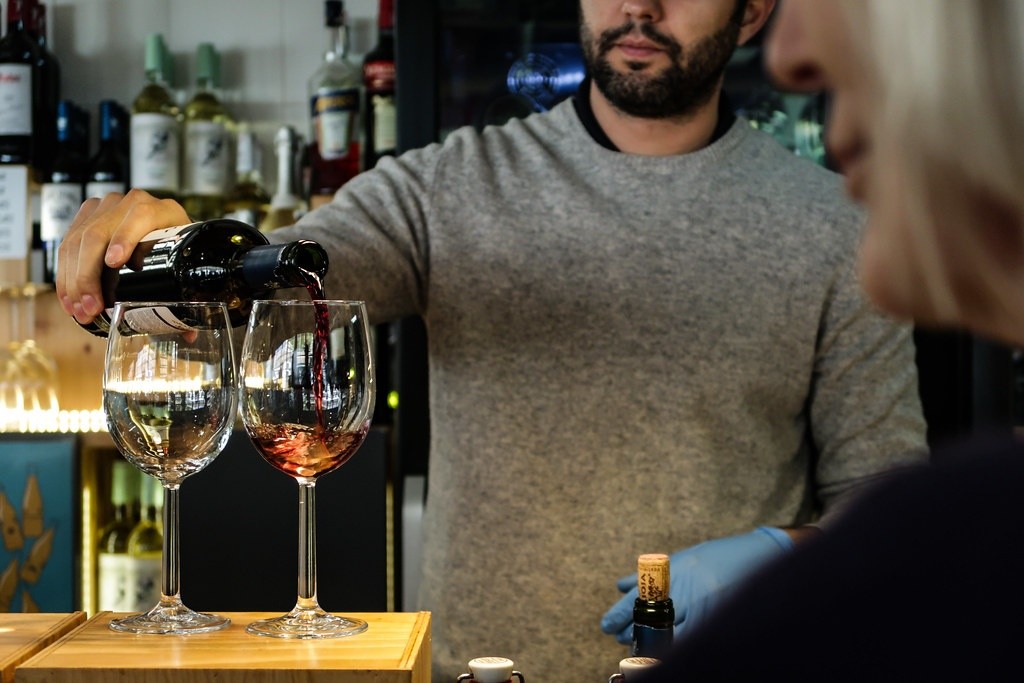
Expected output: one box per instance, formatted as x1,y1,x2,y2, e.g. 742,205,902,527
103,302,238,634
239,300,376,639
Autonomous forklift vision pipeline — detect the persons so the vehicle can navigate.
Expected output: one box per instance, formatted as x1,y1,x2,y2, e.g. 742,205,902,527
628,0,1023,683
55,1,929,683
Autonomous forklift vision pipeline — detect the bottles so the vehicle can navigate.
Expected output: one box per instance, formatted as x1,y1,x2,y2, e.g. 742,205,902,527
130,33,182,204
98,461,166,613
85,100,130,200
309,0,365,195
71,218,330,338
0,1,62,186
40,96,93,283
456,657,525,683
223,120,272,229
257,125,309,234
182,42,237,222
631,553,675,661
608,657,662,683
363,0,398,171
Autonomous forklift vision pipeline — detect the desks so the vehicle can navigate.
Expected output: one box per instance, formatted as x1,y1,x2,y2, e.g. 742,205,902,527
0,609,434,683
